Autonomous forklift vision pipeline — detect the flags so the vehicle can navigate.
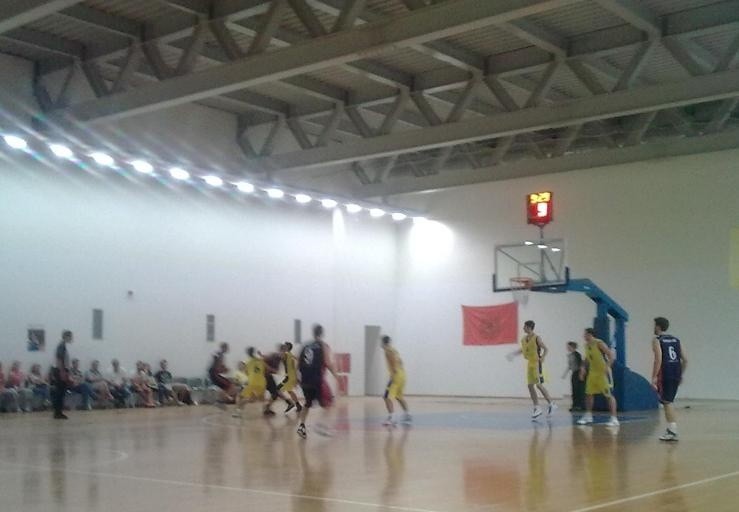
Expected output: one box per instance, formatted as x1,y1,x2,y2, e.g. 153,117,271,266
462,300,519,346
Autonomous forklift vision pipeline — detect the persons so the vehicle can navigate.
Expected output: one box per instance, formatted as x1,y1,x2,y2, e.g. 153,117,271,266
575,327,619,425
504,320,558,418
378,333,414,428
561,339,585,412
649,317,688,442
52,330,75,418
208,342,302,418
70,359,185,408
0,361,52,414
293,324,343,439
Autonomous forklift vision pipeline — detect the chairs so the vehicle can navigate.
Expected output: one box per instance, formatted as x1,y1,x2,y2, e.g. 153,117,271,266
1,373,215,414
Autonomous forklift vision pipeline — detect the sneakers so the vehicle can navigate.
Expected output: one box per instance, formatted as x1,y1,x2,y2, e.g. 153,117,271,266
214,399,228,412
284,403,295,412
2,399,199,413
568,406,584,412
576,412,594,424
263,409,275,416
399,415,413,424
658,428,680,441
531,408,542,421
605,416,620,426
296,425,307,439
231,408,243,417
313,423,337,437
53,410,68,419
382,416,397,425
546,402,558,417
295,405,302,412
